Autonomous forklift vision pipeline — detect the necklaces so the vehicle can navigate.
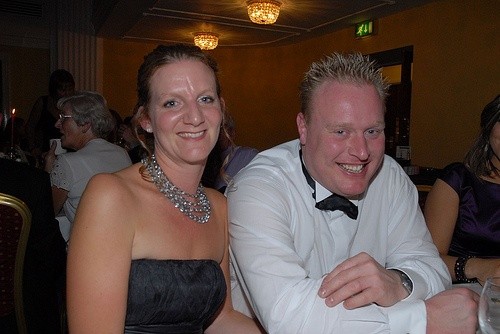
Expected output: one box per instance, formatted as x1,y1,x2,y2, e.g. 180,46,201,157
142,154,211,223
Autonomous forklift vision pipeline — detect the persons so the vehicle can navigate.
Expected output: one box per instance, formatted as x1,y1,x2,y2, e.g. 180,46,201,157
201,124,261,195
424,96,500,284
0,69,155,229
66,44,262,334
224,49,489,334
46,89,131,243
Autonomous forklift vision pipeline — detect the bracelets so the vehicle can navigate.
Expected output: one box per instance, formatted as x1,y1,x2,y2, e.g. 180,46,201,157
455,254,479,284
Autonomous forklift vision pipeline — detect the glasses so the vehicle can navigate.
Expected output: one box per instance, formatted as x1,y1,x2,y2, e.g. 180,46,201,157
58,113,74,123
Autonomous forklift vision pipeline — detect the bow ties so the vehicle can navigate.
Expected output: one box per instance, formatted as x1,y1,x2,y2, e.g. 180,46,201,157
299,148,358,220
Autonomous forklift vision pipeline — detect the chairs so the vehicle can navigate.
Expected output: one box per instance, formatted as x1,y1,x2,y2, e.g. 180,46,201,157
0,193,28,334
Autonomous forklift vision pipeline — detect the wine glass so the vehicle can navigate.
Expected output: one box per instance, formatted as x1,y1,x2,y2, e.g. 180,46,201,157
477,277,500,334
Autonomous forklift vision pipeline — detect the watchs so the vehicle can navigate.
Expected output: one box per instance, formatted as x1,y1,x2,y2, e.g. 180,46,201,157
393,269,413,296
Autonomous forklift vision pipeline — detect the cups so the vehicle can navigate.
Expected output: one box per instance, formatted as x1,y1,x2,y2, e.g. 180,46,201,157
49,138,70,155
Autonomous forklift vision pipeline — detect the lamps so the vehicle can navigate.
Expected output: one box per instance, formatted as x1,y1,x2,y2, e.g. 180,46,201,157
193,32,219,50
245,0,281,24
